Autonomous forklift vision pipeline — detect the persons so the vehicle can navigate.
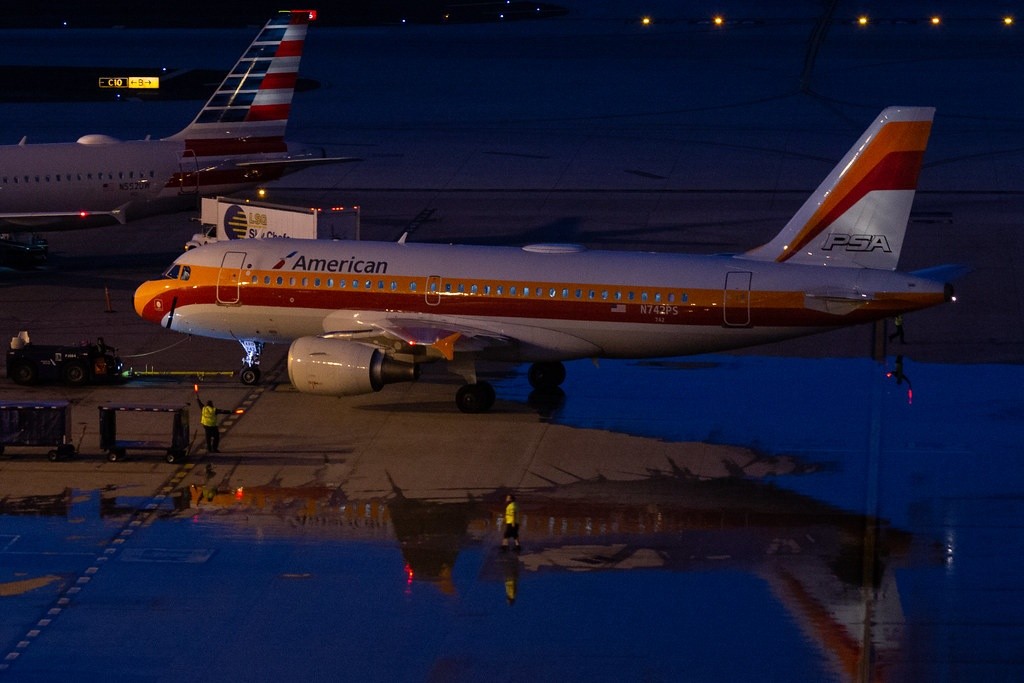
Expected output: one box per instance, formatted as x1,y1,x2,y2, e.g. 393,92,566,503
499,493,521,551
202,485,216,502
195,394,235,452
177,268,190,280
503,571,516,605
31,233,43,241
93,337,115,365
888,313,906,344
889,354,911,389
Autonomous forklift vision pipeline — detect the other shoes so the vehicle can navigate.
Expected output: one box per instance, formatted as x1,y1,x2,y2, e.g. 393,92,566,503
213,448,220,453
207,447,212,452
512,545,521,551
498,545,510,551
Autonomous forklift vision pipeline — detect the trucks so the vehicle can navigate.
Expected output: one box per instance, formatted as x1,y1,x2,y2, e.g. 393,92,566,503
6,330,123,387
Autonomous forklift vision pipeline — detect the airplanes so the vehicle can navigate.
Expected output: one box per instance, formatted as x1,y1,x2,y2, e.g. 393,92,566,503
134,105,946,412
0,10,365,269
